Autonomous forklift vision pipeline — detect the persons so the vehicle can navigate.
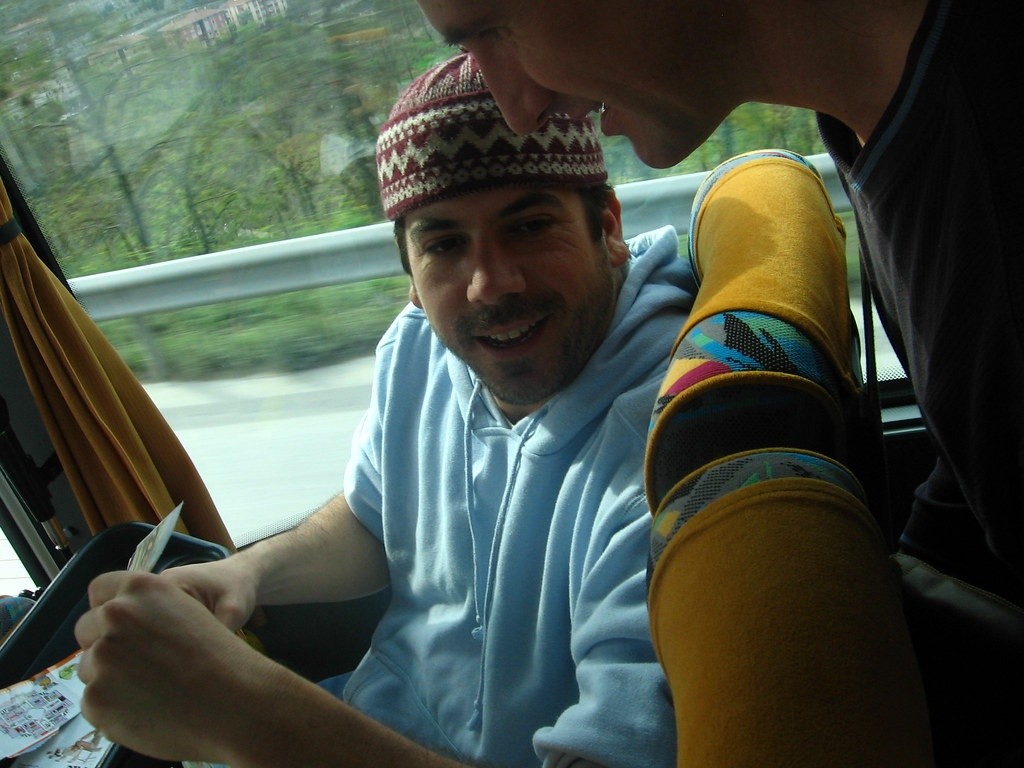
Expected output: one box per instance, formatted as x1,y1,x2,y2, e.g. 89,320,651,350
75,48,698,768
421,0,1024,610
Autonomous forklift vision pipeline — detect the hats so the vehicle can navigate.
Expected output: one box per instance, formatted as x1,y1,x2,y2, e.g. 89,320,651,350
373,46,611,223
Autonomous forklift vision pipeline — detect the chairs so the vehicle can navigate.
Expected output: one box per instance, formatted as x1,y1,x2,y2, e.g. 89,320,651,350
640,147,933,768
